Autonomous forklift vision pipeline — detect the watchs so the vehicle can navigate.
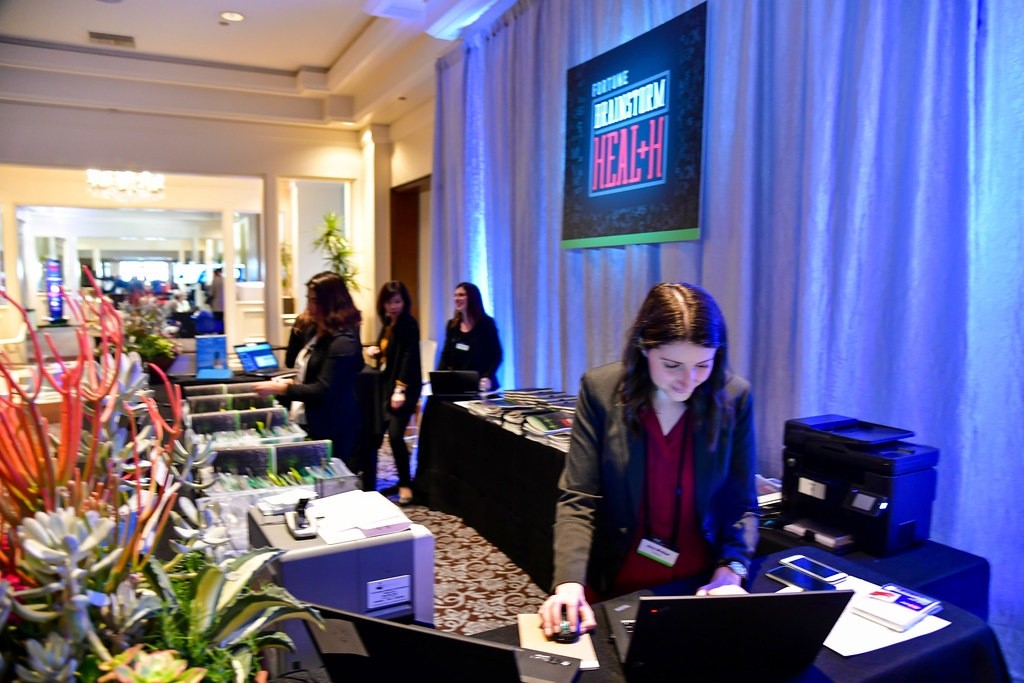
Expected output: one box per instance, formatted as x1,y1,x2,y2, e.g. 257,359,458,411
716,560,749,582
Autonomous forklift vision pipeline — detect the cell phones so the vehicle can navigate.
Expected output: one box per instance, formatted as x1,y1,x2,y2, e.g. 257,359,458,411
779,554,848,586
764,565,836,593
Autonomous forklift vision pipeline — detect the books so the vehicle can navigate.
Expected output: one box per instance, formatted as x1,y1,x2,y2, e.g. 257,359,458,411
851,582,942,632
468,387,578,452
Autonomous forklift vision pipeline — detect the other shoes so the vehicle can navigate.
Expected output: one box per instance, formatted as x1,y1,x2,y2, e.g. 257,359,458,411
399,484,413,504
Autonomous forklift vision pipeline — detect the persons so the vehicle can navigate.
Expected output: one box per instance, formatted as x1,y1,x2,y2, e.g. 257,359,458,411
538,282,760,637
435,282,503,391
108,267,223,319
366,281,421,501
250,271,365,461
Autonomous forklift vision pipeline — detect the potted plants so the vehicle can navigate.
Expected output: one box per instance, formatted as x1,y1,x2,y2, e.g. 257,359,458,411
92,303,183,382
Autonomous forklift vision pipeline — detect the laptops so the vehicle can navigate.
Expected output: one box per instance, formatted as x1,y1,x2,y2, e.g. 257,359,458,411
428,370,480,400
296,601,583,683
601,589,855,683
235,341,299,378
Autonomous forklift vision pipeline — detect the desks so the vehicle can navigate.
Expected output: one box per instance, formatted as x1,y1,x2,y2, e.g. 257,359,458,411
266,546,1011,683
450,396,569,592
